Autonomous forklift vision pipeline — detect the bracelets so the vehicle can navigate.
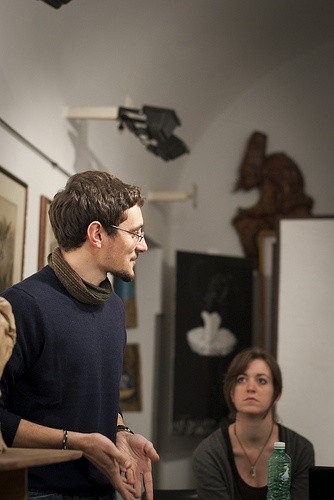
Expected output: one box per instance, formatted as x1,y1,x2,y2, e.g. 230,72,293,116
115,425,136,434
62,429,68,449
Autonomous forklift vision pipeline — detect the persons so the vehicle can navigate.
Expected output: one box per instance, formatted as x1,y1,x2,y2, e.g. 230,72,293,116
0,170,159,500
194,345,319,500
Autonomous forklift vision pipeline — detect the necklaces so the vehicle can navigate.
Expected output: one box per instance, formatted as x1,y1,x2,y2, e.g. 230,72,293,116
231,415,276,478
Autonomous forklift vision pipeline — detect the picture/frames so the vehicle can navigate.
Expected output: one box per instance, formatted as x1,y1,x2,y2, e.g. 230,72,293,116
0,166,27,297
38,195,59,274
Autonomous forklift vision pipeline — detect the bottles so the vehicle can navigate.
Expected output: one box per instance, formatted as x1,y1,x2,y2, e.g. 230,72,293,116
267,442,292,500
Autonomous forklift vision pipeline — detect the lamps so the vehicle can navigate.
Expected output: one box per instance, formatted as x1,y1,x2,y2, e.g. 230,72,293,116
118,102,191,162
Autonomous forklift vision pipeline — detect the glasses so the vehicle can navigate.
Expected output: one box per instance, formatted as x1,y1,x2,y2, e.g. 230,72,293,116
110,222,146,242
